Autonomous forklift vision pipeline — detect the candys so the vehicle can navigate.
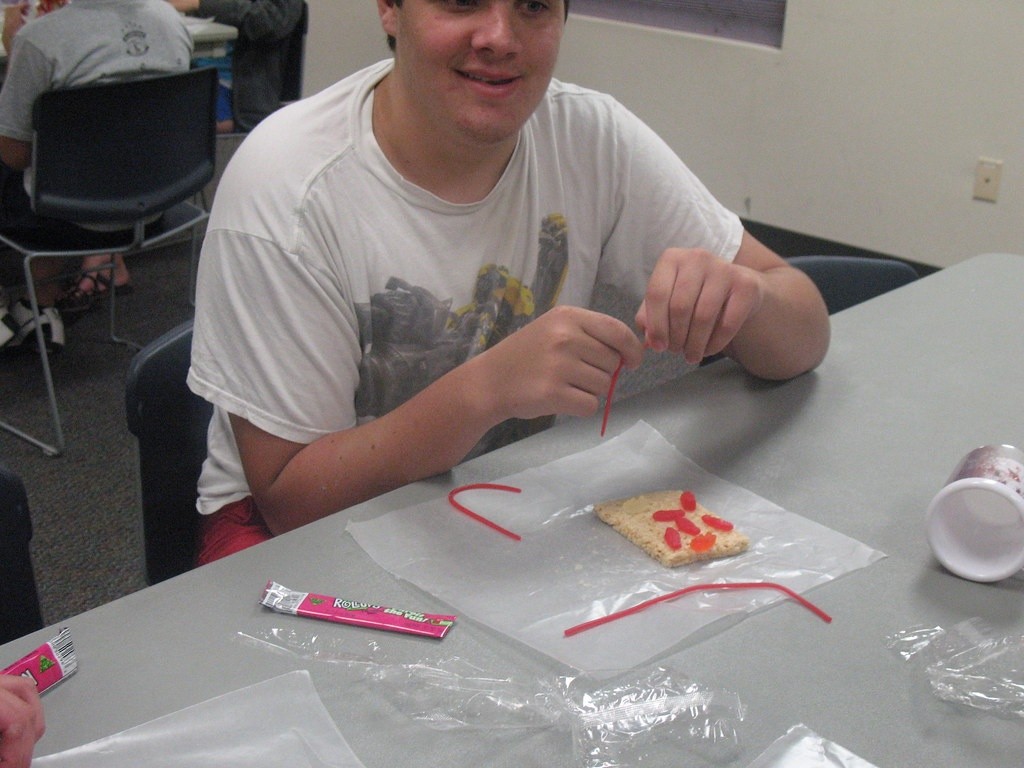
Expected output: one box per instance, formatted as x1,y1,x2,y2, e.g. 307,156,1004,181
652,490,733,553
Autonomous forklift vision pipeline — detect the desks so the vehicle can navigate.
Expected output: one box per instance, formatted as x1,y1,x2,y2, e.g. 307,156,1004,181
0,249,1024,768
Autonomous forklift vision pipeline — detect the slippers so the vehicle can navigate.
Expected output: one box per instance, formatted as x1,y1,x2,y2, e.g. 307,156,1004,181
96,273,136,302
56,271,102,315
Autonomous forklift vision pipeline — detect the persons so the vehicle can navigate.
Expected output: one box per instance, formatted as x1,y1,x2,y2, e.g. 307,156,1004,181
0,0,303,352
0,674,45,768
186,0,831,569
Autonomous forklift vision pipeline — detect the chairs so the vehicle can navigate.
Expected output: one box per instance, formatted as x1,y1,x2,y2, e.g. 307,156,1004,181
123,312,220,588
773,255,916,319
0,65,234,454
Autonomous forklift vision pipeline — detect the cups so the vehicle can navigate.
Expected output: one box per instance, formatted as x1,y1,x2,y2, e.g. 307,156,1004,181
924,444,1024,582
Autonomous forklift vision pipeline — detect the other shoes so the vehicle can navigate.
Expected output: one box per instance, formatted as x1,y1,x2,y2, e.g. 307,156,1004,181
0,298,50,348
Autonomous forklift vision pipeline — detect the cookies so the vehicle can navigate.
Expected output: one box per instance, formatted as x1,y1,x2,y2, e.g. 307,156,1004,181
591,488,751,567
